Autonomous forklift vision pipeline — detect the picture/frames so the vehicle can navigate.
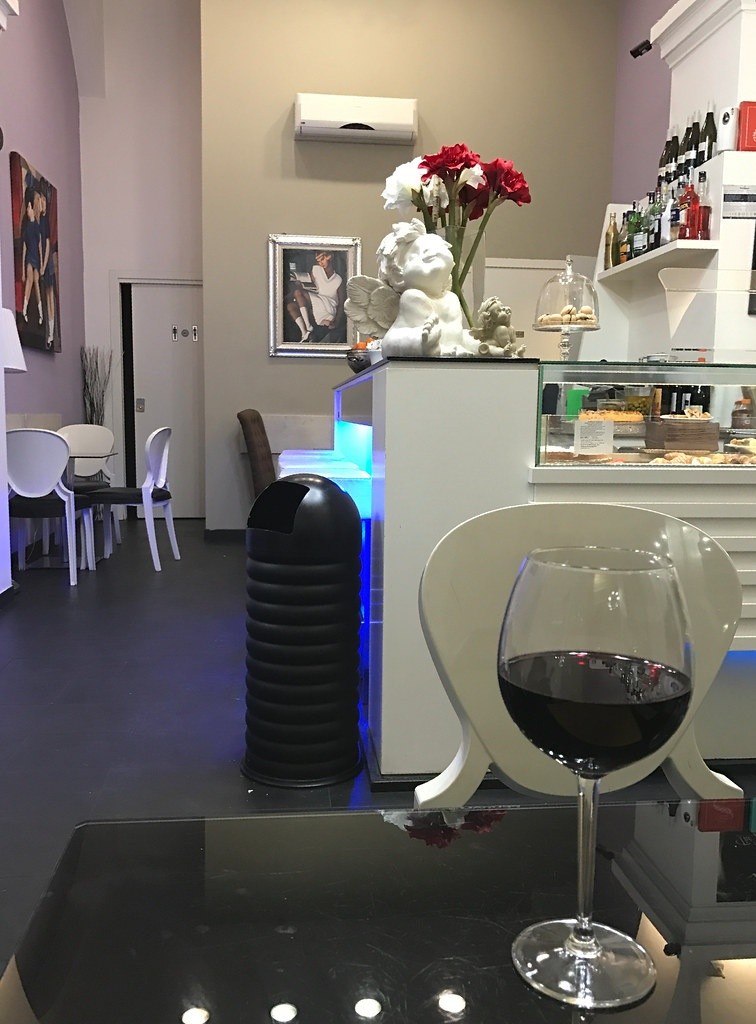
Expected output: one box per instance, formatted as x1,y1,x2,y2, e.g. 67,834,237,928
268,234,362,358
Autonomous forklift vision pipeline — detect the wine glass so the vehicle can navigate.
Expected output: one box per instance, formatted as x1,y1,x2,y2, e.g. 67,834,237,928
496,546,694,1013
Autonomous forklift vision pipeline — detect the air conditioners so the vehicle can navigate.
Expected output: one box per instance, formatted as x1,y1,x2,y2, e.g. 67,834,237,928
294,93,419,145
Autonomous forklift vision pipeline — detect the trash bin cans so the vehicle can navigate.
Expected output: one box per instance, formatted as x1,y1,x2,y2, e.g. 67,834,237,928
236,465,367,793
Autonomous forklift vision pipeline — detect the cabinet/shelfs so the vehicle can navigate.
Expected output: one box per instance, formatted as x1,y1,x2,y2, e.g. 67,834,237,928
574,150,756,363
333,354,756,789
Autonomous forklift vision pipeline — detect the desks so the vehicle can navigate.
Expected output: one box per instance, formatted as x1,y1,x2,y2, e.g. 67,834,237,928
278,450,332,480
32,452,119,571
0,797,756,1024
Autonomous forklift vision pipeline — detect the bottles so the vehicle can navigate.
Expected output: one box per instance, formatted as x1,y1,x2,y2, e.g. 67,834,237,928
604,101,717,269
738,399,751,430
670,357,710,414
731,401,742,429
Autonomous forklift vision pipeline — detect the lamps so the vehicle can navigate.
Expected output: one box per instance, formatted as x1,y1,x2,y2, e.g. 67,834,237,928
3,308,27,593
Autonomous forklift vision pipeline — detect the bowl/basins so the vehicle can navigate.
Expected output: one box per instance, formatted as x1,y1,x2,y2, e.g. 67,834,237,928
346,351,370,374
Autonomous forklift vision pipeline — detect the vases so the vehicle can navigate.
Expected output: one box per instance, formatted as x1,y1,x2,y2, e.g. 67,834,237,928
426,225,486,329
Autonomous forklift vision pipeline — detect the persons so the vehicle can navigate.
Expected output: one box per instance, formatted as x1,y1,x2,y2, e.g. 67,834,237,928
21,186,57,352
343,218,515,356
469,297,527,357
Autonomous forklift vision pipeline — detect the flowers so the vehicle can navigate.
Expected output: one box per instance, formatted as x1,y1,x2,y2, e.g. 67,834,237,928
381,143,532,329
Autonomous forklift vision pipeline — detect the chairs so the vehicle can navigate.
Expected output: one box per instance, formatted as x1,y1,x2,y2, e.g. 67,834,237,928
237,409,277,499
6,428,88,587
85,427,181,571
414,502,744,810
40,424,122,556
